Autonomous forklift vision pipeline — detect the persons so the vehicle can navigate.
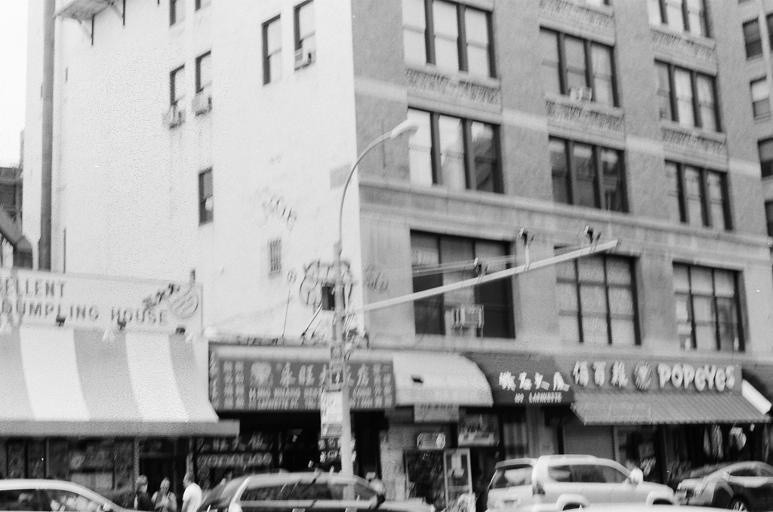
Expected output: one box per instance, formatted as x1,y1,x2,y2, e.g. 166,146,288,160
152,478,178,512
180,472,202,512
133,475,153,512
623,458,644,484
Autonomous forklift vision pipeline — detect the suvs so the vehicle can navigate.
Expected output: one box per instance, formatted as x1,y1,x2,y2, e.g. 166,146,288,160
484,452,679,511
197,470,438,510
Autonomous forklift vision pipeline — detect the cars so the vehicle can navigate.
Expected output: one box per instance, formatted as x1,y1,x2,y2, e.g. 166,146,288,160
0,477,152,511
666,460,772,512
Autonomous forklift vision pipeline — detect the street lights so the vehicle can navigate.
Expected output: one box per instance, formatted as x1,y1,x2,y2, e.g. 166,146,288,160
333,117,419,511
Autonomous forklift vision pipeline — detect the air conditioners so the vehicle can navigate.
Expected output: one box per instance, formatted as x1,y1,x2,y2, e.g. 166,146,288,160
449,304,485,331
